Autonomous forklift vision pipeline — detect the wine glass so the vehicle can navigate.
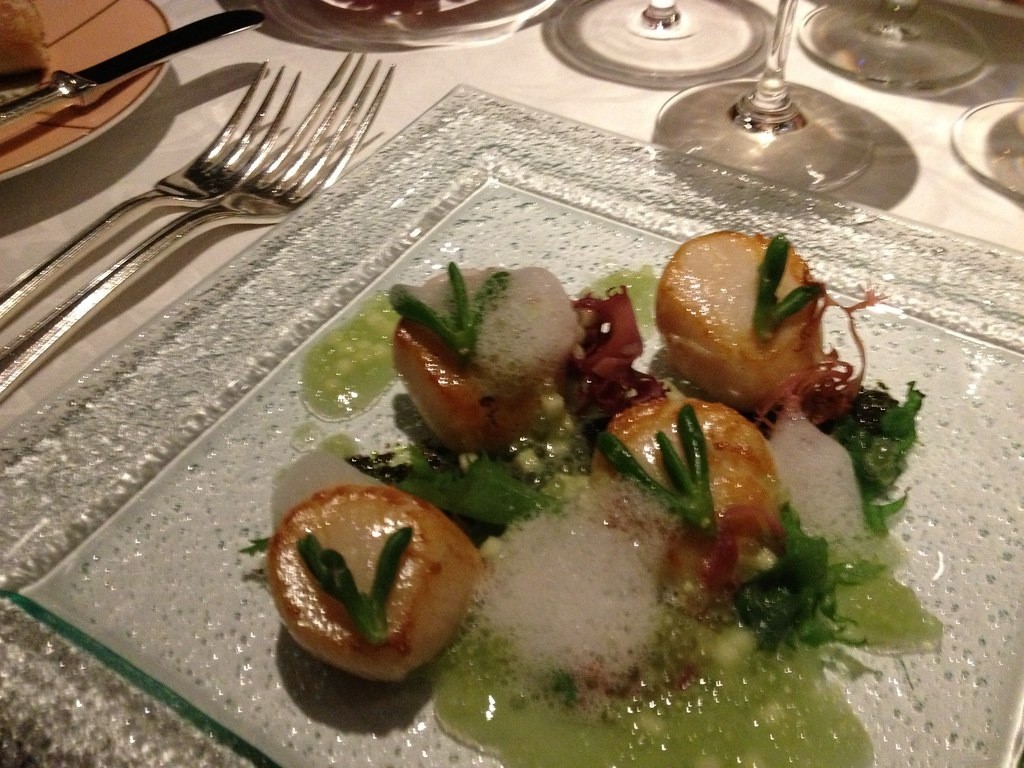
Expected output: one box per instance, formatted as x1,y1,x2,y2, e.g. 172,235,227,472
544,2,1023,211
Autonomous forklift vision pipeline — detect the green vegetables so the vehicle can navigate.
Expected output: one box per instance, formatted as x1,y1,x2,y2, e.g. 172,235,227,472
237,234,925,651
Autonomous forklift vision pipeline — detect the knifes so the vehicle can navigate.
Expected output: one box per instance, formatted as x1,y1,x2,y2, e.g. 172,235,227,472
0,9,265,129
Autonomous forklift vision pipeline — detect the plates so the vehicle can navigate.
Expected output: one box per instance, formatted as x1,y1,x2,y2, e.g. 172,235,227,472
0,0,170,184
0,85,1023,768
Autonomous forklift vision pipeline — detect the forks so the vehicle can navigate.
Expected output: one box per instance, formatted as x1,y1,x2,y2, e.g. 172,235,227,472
0,59,301,326
0,51,396,398
